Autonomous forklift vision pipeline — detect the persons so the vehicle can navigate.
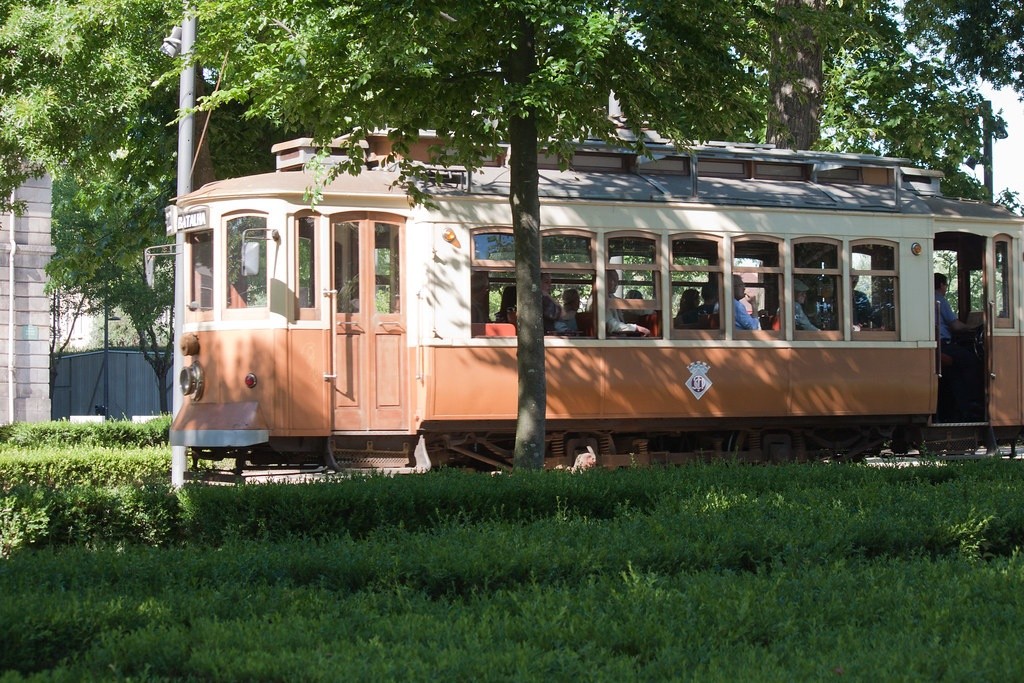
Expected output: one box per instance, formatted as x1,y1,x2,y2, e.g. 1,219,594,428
494,286,516,323
851,268,872,328
776,278,820,331
934,273,984,386
674,275,761,330
541,273,580,332
607,269,650,337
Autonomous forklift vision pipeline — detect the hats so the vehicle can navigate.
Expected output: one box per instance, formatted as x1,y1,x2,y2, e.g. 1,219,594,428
794,279,809,292
562,289,580,310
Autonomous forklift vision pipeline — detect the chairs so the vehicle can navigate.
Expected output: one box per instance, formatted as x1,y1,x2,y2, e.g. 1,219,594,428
473,298,954,341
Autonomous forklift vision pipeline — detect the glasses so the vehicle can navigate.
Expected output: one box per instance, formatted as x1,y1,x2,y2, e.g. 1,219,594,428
733,283,745,288
943,283,948,287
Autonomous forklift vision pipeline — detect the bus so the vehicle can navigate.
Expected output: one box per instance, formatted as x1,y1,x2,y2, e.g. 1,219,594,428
168,118,1024,474
